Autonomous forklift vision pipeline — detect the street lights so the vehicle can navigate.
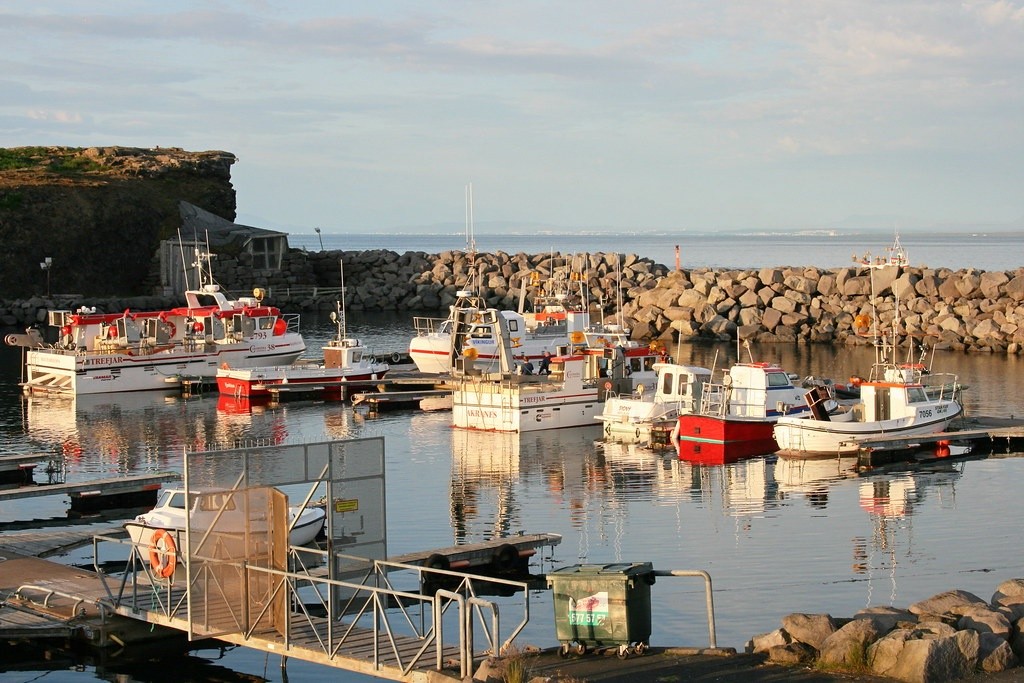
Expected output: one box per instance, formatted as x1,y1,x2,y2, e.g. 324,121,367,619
314,227,324,251
40,257,53,300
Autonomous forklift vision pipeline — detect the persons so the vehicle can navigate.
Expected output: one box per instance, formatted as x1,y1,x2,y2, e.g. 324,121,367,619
539,352,556,375
520,356,534,375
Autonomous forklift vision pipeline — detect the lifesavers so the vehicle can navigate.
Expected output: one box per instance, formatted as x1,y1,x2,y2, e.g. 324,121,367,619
166,322,176,339
464,348,478,360
752,362,770,368
423,553,450,584
571,332,584,343
369,354,377,364
494,543,520,573
148,528,177,579
650,340,658,350
392,352,401,363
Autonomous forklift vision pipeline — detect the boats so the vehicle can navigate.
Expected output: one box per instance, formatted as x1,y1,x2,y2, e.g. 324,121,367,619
771,255,964,456
215,260,391,398
863,232,912,268
450,253,717,433
4,226,306,398
123,487,326,565
675,326,849,464
593,321,733,442
407,183,639,375
